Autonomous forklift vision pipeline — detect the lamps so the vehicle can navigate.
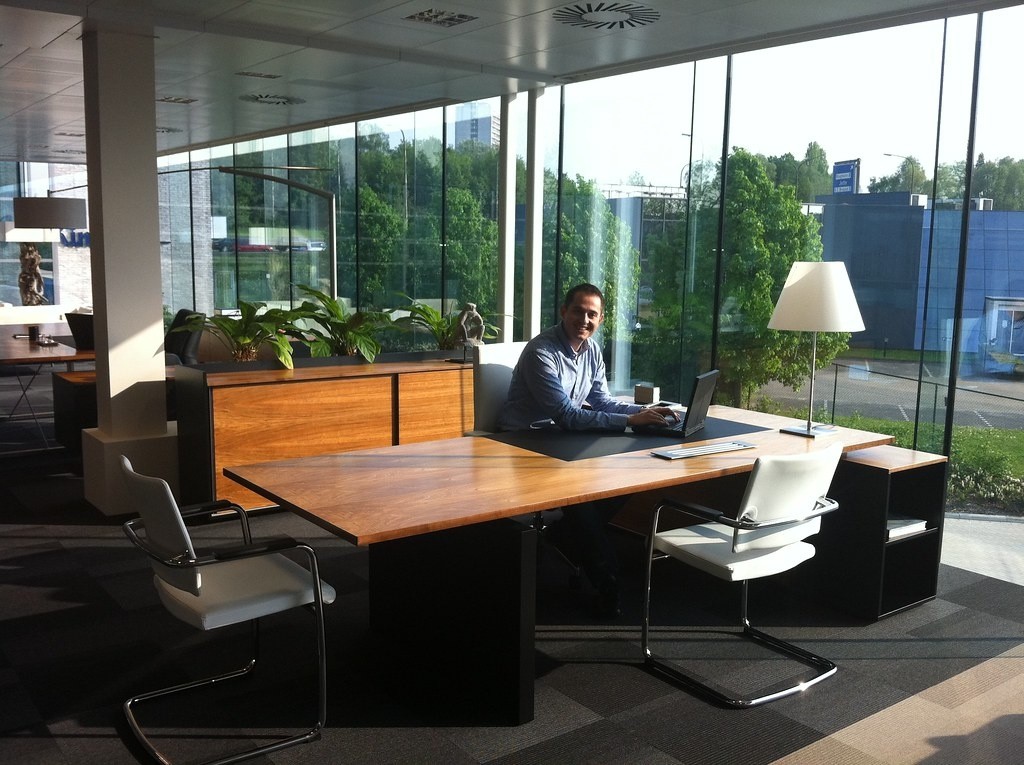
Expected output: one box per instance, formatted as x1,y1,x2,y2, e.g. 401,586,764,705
766,261,867,438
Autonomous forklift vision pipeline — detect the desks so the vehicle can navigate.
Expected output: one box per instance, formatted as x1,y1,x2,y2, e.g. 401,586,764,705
0,322,97,459
223,404,894,728
175,349,473,517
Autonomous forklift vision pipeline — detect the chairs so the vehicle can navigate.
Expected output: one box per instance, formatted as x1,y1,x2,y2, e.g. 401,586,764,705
642,442,843,711
165,309,205,365
65,314,93,352
117,454,336,765
474,340,583,590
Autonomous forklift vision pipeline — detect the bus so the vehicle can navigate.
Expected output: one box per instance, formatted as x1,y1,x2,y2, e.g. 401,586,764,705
978,293,1024,379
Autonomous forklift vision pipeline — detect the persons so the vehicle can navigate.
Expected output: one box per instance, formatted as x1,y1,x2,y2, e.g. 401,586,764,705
499,283,682,620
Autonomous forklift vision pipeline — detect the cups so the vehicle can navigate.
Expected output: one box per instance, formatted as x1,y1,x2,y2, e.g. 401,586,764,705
29,326,39,343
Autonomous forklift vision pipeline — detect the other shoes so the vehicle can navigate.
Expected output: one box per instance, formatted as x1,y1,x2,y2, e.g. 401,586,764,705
597,574,623,619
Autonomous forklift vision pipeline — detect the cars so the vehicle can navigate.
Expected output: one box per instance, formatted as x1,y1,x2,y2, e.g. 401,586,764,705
211,235,326,252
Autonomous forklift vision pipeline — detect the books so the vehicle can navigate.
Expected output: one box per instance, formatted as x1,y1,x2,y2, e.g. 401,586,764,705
886,512,928,542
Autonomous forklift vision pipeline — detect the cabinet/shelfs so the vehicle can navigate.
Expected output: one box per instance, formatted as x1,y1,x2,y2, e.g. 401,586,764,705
811,446,950,625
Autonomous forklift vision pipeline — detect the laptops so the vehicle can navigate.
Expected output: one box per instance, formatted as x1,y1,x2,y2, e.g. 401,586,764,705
632,370,719,437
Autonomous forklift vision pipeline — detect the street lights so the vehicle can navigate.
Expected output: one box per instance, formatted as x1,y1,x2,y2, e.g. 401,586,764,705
883,153,914,194
